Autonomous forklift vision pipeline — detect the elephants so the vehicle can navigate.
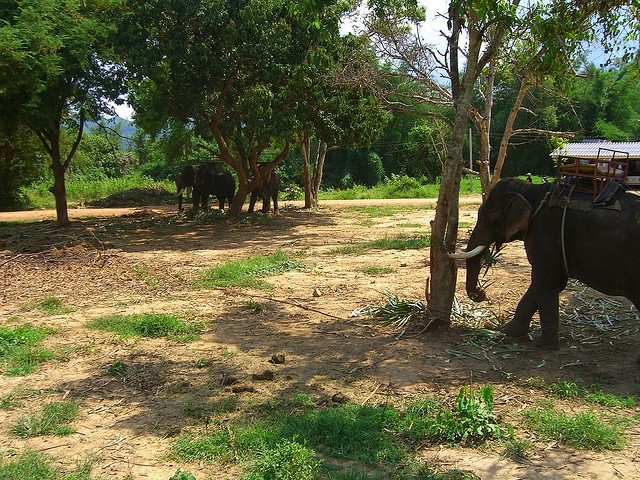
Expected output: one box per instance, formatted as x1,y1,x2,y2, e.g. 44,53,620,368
175,163,236,210
447,175,640,349
247,167,279,211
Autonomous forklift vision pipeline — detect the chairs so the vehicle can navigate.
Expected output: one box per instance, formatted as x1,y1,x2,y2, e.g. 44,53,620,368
556,147,630,201
